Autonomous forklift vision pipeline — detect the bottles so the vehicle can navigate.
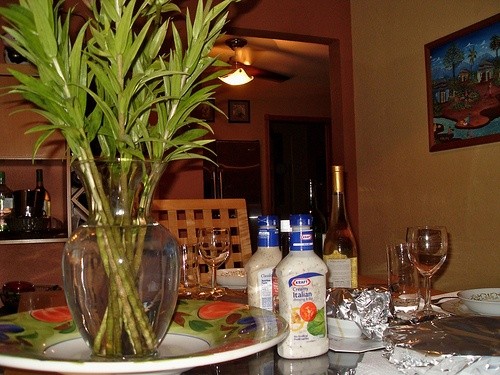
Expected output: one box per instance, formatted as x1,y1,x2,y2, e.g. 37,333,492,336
33,168,50,217
323,164,357,290
247,352,275,375
300,176,326,259
0,171,14,231
272,213,330,359
277,354,329,375
245,216,283,310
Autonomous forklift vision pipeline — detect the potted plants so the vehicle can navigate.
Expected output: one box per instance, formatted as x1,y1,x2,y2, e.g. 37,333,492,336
0,0,241,360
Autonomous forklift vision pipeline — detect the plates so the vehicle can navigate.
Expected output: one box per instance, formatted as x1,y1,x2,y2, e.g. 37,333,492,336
0,300,290,375
441,298,471,314
328,337,386,352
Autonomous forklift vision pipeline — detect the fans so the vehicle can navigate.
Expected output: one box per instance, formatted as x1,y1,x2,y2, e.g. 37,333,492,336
208,38,293,84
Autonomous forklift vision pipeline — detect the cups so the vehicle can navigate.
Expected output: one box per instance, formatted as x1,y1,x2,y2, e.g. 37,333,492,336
385,242,420,313
177,242,200,295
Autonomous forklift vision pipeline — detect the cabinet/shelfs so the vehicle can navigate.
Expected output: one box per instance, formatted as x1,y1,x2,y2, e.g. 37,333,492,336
0,0,159,244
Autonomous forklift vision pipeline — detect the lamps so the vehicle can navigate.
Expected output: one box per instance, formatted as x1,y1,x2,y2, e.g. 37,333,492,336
217,66,254,86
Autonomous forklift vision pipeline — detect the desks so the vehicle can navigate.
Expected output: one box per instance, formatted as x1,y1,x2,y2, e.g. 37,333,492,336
0,289,500,375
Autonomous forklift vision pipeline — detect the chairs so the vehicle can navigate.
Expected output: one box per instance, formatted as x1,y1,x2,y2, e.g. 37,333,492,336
148,198,252,286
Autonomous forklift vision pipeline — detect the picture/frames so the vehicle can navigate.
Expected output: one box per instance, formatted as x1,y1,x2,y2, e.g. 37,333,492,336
227,100,250,124
190,99,216,122
424,13,500,152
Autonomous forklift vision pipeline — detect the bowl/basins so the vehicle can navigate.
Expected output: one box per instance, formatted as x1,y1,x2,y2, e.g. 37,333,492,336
5,188,50,231
326,317,362,339
216,268,247,290
328,351,364,368
0,281,36,311
457,288,500,316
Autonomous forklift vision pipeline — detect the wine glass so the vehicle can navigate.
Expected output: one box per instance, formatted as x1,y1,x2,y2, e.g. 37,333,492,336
406,225,448,318
197,227,231,298
